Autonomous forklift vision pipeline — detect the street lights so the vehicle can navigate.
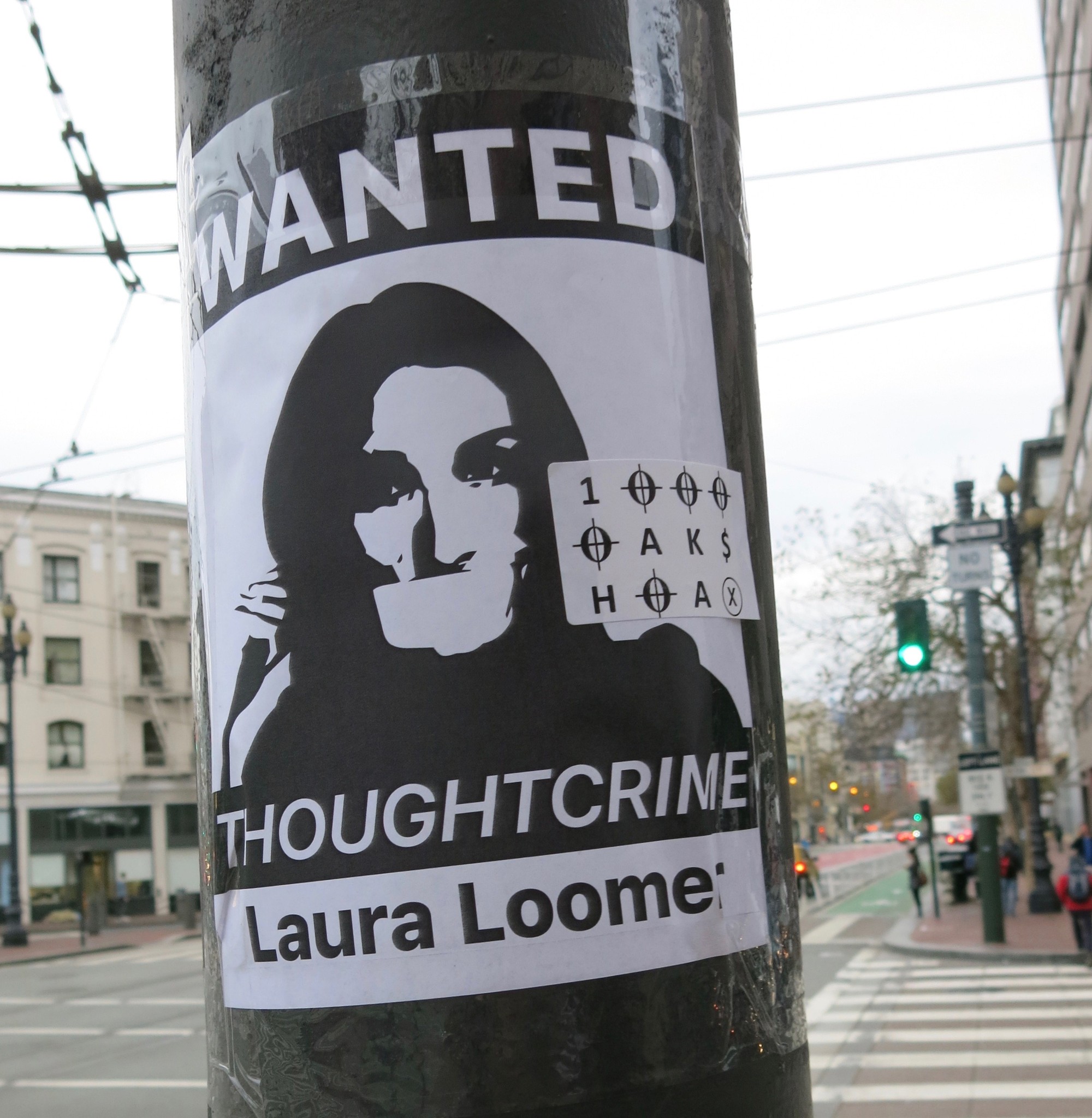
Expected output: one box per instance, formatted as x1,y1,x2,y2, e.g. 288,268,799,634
1,592,32,944
980,463,1064,912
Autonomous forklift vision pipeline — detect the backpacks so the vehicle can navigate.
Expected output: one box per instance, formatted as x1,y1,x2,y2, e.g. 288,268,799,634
999,856,1012,877
1068,868,1089,902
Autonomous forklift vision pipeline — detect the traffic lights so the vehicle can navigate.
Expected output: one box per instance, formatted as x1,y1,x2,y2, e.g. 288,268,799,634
893,600,933,673
920,799,930,818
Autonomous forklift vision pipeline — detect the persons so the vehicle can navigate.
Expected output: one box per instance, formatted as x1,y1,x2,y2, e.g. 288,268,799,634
116,873,129,921
965,841,982,905
1071,824,1092,865
1053,818,1063,852
1056,856,1092,967
998,829,1026,918
906,846,928,917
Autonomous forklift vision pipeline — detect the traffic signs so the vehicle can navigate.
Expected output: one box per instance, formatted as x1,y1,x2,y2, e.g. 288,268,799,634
930,518,1006,548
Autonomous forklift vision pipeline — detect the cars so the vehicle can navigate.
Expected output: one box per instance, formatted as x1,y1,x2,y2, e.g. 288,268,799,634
931,813,971,864
854,831,895,843
793,845,820,897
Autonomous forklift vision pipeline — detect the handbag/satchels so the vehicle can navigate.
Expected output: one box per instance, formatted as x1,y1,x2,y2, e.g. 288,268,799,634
917,874,926,886
